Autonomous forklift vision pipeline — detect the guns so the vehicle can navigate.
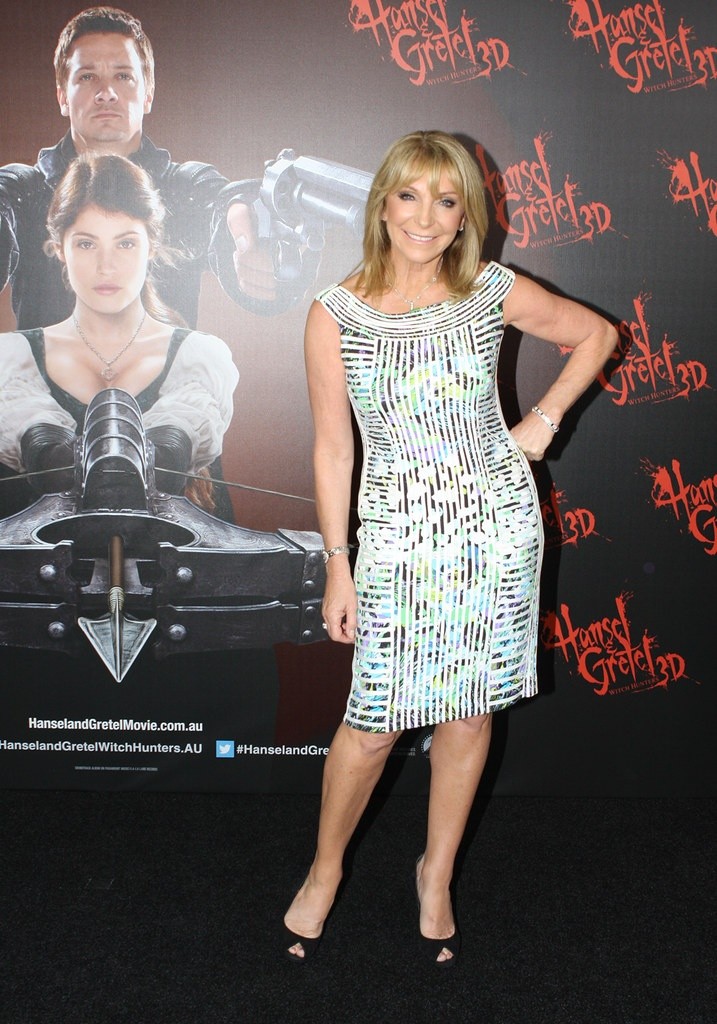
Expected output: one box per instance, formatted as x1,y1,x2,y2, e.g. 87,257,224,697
243,145,376,292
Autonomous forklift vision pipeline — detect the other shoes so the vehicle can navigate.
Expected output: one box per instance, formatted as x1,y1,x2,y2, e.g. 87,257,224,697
280,891,324,961
414,894,460,969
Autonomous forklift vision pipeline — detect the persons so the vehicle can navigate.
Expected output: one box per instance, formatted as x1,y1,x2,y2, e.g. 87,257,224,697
271,128,622,968
0,6,322,524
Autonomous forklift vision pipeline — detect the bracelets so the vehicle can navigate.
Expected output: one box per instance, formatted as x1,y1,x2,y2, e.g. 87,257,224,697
322,546,350,564
530,405,560,434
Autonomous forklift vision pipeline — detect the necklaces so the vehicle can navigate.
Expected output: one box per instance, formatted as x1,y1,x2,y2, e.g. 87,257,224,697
381,257,443,312
